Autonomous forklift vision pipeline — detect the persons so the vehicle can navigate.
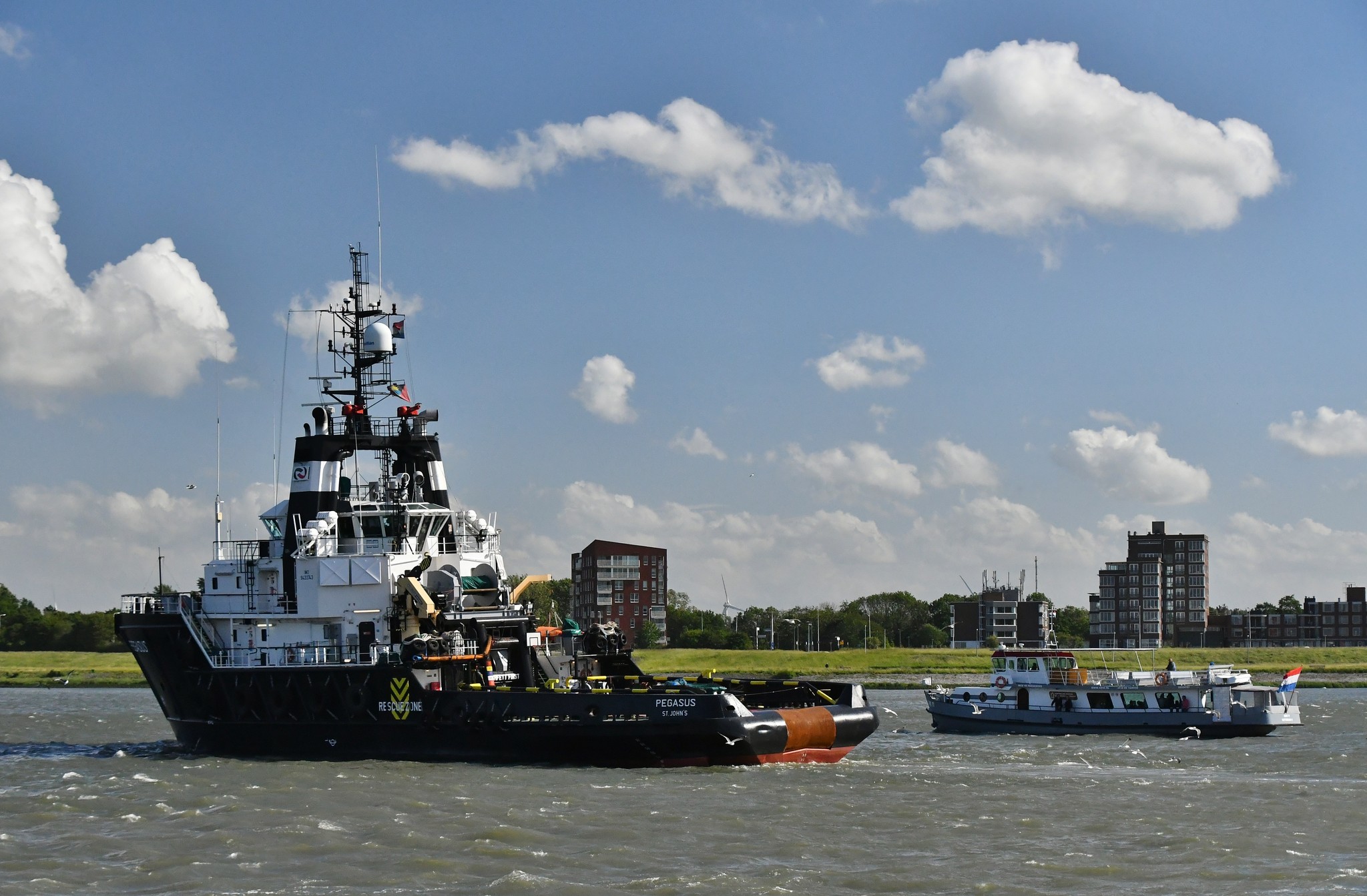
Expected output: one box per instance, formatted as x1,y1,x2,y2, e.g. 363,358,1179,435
1210,659,1214,670
1064,694,1072,712
1165,658,1176,685
1229,667,1232,674
1181,696,1190,713
1158,693,1180,709
1127,698,1148,709
1051,694,1063,712
1030,663,1037,672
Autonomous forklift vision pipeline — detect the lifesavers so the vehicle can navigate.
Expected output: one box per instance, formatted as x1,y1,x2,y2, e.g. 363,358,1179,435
607,634,619,646
1155,673,1167,686
595,636,608,649
618,634,627,646
581,669,593,676
413,639,427,652
284,648,294,663
438,639,449,652
996,676,1006,688
427,638,439,651
581,631,584,635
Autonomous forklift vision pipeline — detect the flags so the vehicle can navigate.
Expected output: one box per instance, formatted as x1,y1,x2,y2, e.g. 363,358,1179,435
388,383,411,403
393,320,405,338
1276,666,1302,693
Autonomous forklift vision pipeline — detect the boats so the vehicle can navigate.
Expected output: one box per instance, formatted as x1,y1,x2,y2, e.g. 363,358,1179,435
110,237,884,772
921,558,1305,739
